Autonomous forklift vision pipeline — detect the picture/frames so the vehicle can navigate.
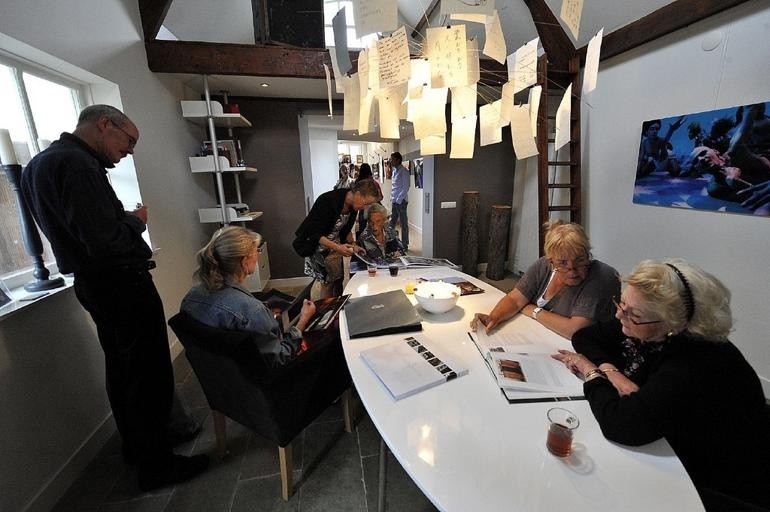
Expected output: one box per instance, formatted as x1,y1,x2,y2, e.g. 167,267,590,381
338,152,425,194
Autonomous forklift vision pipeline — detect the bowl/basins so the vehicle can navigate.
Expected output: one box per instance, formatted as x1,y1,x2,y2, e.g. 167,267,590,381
413,291,459,313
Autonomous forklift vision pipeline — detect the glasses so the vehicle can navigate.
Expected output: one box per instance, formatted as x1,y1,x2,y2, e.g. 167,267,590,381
611,295,665,325
548,254,589,273
110,120,137,146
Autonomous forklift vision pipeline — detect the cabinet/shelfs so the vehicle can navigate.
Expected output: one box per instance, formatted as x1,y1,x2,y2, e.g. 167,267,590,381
178,94,276,292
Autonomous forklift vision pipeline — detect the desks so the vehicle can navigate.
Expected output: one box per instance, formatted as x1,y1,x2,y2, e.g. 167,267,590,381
335,250,710,512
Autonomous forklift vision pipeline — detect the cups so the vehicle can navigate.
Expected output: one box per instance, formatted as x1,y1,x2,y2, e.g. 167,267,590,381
223,103,240,114
405,280,414,294
389,262,399,278
546,408,579,457
367,263,377,278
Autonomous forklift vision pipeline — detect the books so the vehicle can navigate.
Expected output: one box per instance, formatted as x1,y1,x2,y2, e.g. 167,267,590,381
468,317,588,404
349,250,460,275
420,277,485,296
359,334,469,399
257,288,351,335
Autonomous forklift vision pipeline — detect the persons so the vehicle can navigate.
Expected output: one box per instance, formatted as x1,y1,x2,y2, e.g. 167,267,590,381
636,103,770,202
292,150,410,299
470,219,621,340
552,257,769,511
181,226,339,371
19,105,202,492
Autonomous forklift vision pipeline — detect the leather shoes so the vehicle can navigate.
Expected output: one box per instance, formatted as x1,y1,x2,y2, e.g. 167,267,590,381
158,454,207,483
171,421,201,443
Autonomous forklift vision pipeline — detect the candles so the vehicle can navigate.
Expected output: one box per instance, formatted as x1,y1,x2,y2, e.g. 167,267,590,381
0,125,17,166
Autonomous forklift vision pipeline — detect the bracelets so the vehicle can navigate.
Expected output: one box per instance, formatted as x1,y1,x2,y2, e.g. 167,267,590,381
585,369,600,378
603,369,621,372
533,307,542,318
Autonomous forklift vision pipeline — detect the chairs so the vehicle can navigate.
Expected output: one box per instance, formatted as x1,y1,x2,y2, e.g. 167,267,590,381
161,300,355,503
